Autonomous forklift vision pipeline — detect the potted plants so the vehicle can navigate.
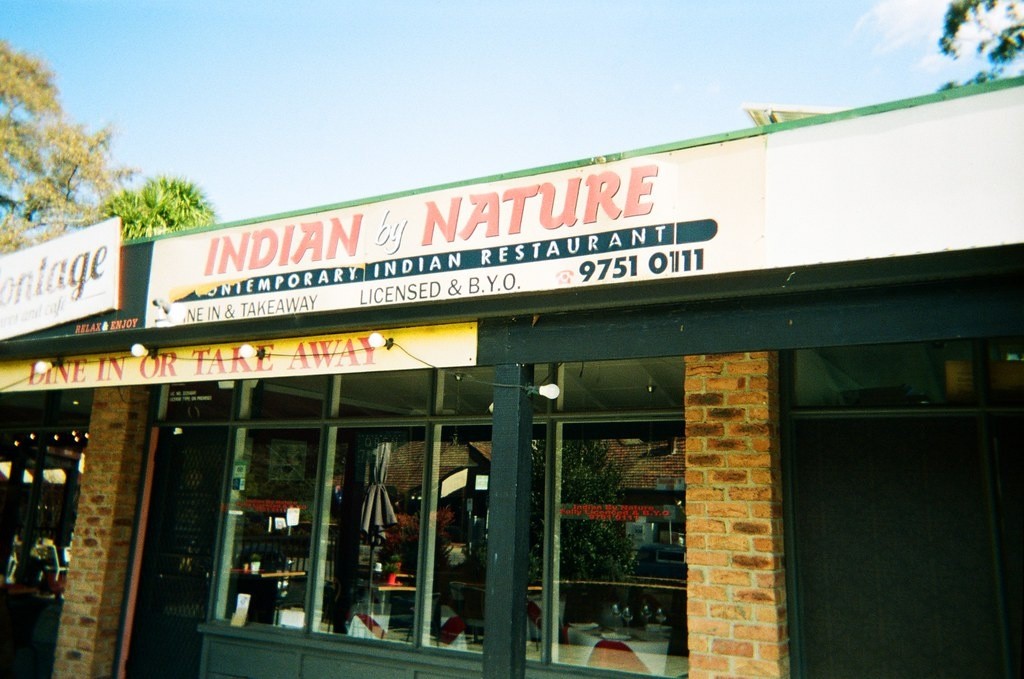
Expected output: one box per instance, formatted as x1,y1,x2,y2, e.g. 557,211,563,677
391,555,402,572
381,562,399,584
251,553,262,571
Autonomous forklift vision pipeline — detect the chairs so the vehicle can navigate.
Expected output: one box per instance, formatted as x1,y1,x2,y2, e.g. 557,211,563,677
404,593,443,647
34,544,68,598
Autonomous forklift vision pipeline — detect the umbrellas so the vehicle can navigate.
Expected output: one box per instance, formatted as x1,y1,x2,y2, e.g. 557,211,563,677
359,441,398,628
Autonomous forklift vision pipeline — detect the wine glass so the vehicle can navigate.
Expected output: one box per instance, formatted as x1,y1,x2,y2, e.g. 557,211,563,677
640,602,653,642
611,603,633,639
655,607,666,641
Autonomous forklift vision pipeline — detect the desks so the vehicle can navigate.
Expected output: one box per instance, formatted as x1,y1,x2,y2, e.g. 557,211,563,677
450,581,543,644
375,574,414,614
230,568,307,626
372,585,417,640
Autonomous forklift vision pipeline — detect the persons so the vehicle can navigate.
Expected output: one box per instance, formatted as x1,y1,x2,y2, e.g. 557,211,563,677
331,485,341,518
